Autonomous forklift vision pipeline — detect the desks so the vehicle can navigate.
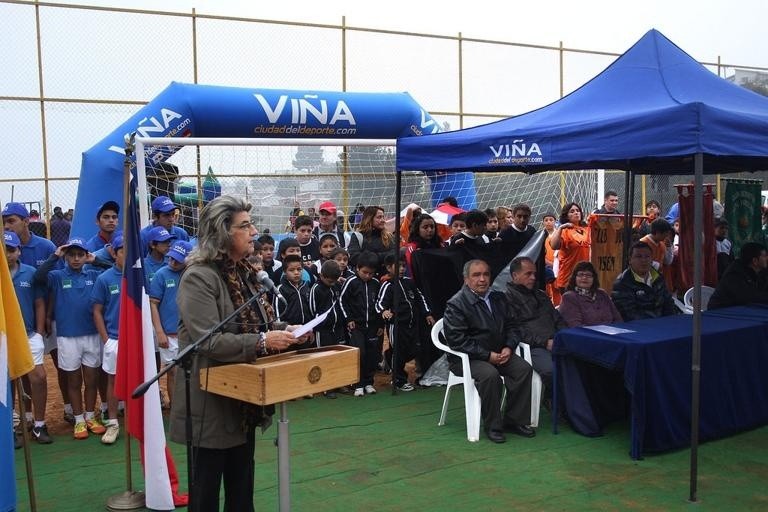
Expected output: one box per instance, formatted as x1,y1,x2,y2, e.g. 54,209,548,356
552,306,768,461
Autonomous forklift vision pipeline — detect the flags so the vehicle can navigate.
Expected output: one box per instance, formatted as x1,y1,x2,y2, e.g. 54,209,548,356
114,175,188,512
0,241,35,510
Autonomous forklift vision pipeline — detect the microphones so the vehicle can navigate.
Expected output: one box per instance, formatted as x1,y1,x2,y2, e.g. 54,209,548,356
255,269,288,306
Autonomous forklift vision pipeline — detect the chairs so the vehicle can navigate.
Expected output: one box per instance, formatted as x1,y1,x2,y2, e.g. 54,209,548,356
519,342,543,428
673,297,692,314
430,317,521,443
684,286,715,312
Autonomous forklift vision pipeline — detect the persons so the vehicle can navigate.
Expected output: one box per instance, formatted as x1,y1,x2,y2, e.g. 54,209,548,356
707,190,768,311
170,195,315,512
253,194,469,397
0,196,197,446
443,191,681,441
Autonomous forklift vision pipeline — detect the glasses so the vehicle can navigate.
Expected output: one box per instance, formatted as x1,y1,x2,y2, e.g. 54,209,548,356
577,272,593,278
232,220,255,231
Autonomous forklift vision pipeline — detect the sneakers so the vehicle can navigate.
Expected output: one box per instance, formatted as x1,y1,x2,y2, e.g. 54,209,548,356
64,408,119,444
366,384,377,395
354,387,365,397
397,383,414,391
31,425,52,444
327,391,336,399
14,419,34,449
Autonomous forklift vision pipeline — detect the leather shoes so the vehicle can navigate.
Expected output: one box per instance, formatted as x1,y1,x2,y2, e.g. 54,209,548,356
509,424,536,437
484,427,505,443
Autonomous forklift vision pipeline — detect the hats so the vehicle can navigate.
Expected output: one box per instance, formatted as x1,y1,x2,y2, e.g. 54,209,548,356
1,202,28,218
4,231,22,248
96,200,120,219
319,202,336,214
149,196,193,264
112,236,123,250
66,237,88,251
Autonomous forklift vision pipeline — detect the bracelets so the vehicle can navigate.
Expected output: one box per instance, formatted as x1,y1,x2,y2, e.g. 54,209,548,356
258,330,269,356
